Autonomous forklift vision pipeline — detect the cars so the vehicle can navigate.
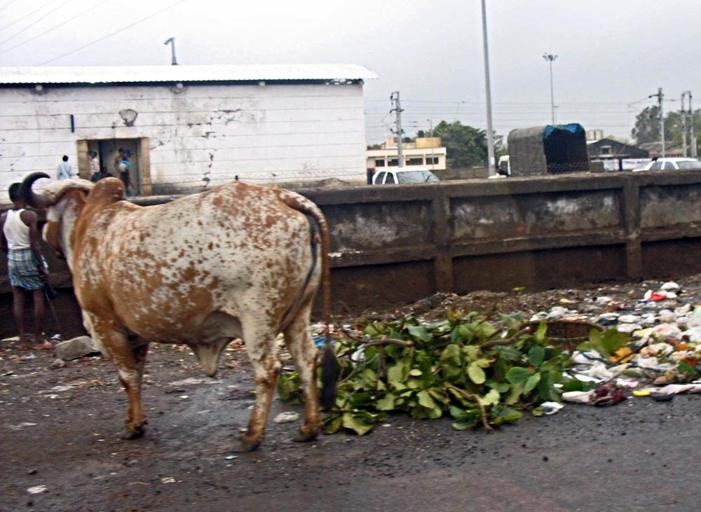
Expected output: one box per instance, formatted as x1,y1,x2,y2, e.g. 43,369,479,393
630,156,701,173
370,165,440,185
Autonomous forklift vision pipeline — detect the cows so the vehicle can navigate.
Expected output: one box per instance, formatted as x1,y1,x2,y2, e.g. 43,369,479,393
18,171,341,457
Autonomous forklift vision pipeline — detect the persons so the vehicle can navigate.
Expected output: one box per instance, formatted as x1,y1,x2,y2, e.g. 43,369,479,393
55,154,75,180
87,151,102,182
113,146,133,198
0,181,56,350
100,166,112,177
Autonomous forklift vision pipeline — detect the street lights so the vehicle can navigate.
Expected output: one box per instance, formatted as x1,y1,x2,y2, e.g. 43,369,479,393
541,52,559,125
647,93,665,158
